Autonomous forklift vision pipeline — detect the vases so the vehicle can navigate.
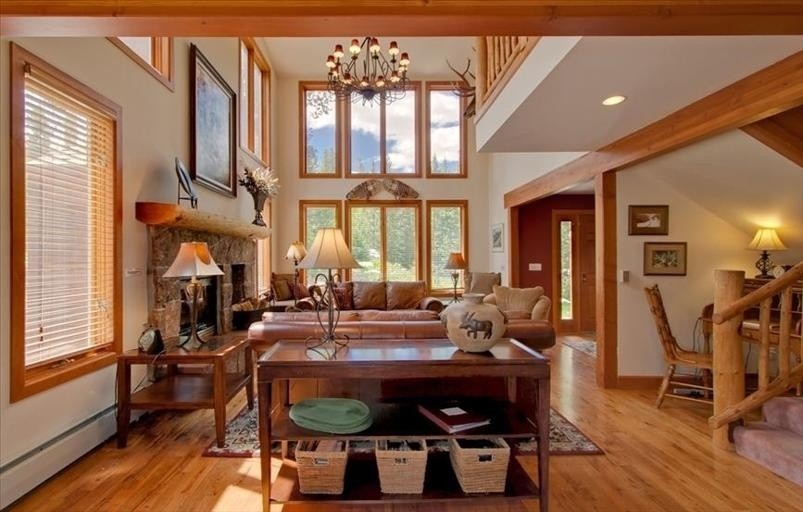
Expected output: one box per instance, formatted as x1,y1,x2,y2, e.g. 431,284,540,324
250,191,266,226
440,294,507,353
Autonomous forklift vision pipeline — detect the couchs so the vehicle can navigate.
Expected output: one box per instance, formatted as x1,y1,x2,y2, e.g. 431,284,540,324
248,321,556,423
484,285,552,319
265,272,302,312
296,281,445,322
466,271,501,294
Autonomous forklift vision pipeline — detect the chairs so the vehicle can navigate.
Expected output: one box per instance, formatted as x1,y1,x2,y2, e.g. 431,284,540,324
643,284,712,409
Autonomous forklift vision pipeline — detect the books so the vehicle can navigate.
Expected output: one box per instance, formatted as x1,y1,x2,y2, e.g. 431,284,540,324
419,399,491,435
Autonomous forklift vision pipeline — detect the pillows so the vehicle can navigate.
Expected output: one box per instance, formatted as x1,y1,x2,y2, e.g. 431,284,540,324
288,282,310,299
273,280,292,301
332,287,348,310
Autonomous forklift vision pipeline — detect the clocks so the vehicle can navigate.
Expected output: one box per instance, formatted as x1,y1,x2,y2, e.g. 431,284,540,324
774,266,785,279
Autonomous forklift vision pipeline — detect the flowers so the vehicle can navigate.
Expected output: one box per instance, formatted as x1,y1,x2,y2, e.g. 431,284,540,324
238,165,282,199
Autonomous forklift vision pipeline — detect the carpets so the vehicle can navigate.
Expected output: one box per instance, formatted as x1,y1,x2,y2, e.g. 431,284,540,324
202,393,606,455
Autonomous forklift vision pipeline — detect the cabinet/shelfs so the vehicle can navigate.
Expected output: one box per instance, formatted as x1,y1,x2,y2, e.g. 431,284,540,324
256,338,550,512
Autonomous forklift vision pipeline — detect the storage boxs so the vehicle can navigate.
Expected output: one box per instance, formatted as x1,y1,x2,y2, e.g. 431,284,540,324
448,438,511,494
295,439,349,494
375,439,428,494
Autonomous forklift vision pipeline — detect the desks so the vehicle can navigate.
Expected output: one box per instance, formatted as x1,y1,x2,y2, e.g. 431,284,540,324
702,278,803,400
116,335,255,448
439,297,464,305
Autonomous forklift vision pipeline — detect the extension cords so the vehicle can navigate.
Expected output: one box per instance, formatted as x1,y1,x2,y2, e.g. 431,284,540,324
674,388,705,396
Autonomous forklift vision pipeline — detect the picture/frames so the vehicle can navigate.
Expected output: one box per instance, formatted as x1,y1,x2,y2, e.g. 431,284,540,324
629,205,669,235
190,43,237,199
491,224,504,252
644,243,687,276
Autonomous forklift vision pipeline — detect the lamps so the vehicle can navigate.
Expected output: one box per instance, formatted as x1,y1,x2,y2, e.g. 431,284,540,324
163,242,225,349
325,37,411,108
746,229,787,279
443,253,467,304
294,227,363,355
284,241,307,284
